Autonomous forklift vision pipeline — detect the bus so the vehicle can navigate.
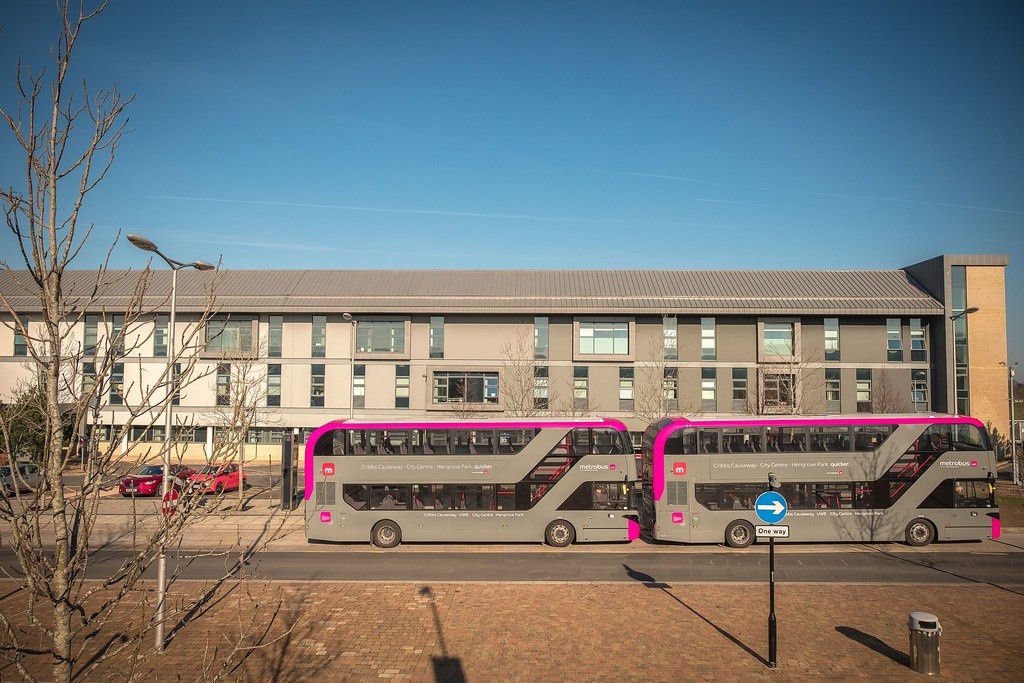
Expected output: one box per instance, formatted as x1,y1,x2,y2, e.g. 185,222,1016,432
638,411,1002,549
303,419,640,548
820,549,822,550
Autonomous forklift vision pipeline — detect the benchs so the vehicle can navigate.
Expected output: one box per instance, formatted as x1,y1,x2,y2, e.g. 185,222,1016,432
344,485,367,509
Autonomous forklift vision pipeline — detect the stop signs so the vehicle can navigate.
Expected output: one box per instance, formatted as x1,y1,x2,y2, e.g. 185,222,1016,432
161,489,180,517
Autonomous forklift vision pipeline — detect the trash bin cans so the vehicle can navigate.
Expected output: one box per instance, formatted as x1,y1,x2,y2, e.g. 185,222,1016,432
906,611,942,676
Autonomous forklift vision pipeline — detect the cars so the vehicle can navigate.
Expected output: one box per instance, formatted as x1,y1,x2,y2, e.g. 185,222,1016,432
0,461,43,498
118,463,198,497
184,461,248,495
127,232,215,653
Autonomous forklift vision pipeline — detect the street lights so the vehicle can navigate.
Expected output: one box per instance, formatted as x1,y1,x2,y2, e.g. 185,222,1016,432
948,306,979,482
767,471,781,669
342,312,356,445
999,359,1018,483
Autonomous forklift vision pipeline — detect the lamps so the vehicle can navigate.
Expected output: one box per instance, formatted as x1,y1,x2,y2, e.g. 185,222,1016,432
342,313,355,326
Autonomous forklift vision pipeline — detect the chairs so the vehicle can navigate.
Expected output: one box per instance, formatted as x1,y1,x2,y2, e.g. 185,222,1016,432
699,434,848,453
744,498,754,509
381,489,468,510
333,437,516,454
